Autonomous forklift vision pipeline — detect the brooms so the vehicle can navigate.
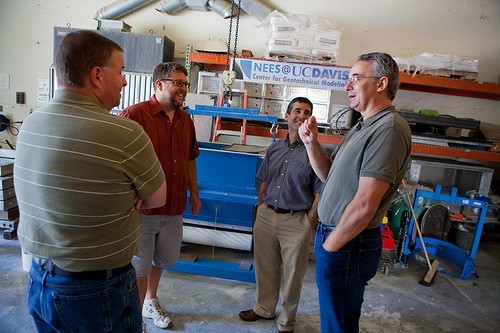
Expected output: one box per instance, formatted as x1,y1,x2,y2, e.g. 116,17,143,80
402,178,439,287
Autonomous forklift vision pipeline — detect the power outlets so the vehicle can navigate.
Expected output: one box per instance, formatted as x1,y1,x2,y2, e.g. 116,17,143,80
29,108,34,114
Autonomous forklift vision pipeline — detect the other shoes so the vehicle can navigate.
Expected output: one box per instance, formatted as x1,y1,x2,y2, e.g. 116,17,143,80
239,309,276,321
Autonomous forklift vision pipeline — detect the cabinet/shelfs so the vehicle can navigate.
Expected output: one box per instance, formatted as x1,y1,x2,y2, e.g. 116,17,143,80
185,44,500,162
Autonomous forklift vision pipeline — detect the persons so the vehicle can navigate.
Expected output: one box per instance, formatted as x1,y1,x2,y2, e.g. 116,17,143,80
239,97,322,333
298,53,411,333
120,62,202,328
13,30,166,333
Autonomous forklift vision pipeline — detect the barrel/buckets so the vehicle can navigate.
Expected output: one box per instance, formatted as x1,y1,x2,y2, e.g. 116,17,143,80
452,224,476,250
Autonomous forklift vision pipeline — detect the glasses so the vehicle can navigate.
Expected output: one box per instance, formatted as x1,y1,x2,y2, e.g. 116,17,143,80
346,76,382,84
158,79,190,89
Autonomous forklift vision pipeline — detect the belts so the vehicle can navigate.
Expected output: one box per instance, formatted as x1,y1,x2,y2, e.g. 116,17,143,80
316,222,336,232
267,203,307,215
31,256,135,280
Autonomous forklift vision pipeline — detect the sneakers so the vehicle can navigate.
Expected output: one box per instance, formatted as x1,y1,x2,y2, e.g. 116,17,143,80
142,298,172,329
